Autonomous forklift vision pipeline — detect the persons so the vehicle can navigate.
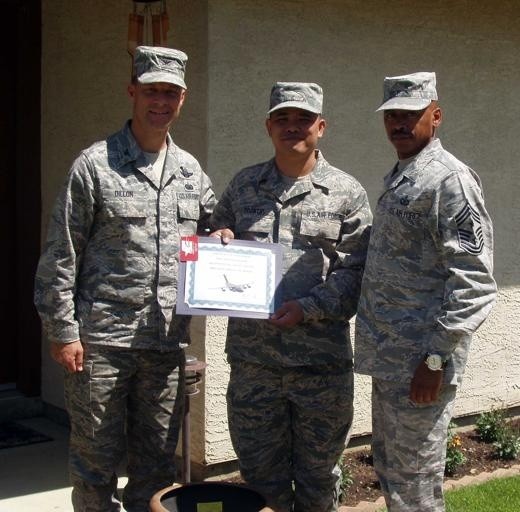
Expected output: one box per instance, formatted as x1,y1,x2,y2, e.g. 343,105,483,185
31,43,217,511
206,80,373,512
351,68,495,512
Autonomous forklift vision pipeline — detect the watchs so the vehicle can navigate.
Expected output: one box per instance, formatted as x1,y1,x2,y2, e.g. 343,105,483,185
418,349,449,371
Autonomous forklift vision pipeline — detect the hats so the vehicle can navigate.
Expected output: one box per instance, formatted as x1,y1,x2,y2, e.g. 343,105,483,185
132,46,188,89
374,71,438,114
267,81,324,116
